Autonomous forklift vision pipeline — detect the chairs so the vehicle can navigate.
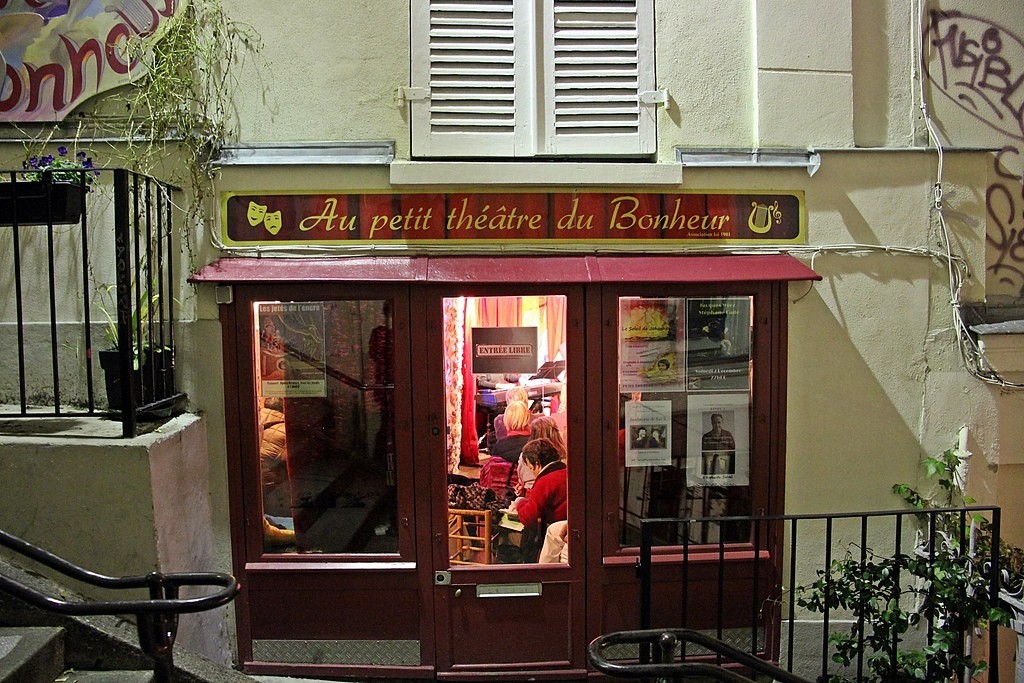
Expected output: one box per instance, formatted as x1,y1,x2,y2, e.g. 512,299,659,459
447,507,552,566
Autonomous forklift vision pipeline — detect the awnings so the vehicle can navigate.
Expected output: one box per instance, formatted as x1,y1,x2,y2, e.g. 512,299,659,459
187,250,825,293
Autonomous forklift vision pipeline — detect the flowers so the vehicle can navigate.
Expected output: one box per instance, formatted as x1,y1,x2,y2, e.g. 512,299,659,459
19,146,100,186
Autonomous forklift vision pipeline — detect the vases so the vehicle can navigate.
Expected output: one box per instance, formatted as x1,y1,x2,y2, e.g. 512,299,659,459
0,180,87,228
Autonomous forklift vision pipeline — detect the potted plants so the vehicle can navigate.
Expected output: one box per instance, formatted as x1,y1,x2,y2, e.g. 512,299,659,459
92,250,178,423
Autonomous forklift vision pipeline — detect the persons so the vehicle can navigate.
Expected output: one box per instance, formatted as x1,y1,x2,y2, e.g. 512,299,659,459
260,396,290,494
633,427,666,449
476,369,568,564
701,413,735,450
